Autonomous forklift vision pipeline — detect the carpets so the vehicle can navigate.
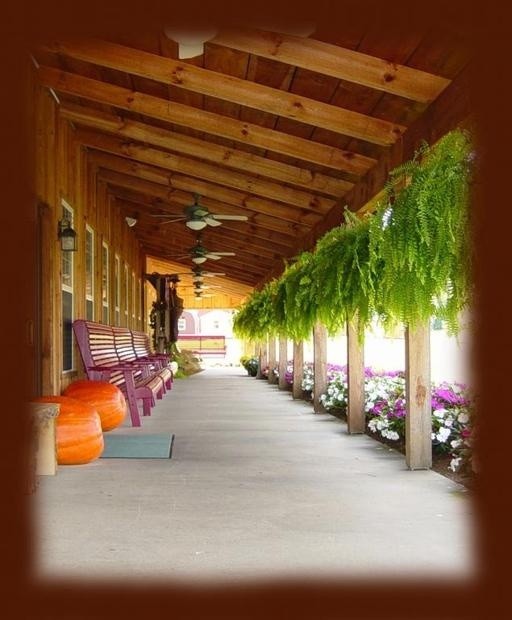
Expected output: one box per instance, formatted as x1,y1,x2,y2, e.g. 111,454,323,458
99,433,175,459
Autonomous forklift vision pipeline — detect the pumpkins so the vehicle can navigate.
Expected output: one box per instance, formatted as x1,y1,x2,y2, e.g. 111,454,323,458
31,395,104,464
63,379,128,431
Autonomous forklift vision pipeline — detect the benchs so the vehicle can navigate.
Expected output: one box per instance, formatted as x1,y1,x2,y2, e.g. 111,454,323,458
71,319,179,427
175,334,227,361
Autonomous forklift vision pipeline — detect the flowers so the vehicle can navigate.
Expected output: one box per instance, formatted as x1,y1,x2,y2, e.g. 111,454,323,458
239,351,479,475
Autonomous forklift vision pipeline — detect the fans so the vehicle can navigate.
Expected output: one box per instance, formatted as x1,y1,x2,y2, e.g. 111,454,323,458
150,193,248,282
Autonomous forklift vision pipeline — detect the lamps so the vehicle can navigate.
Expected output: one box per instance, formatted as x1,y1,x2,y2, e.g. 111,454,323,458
58,216,78,251
